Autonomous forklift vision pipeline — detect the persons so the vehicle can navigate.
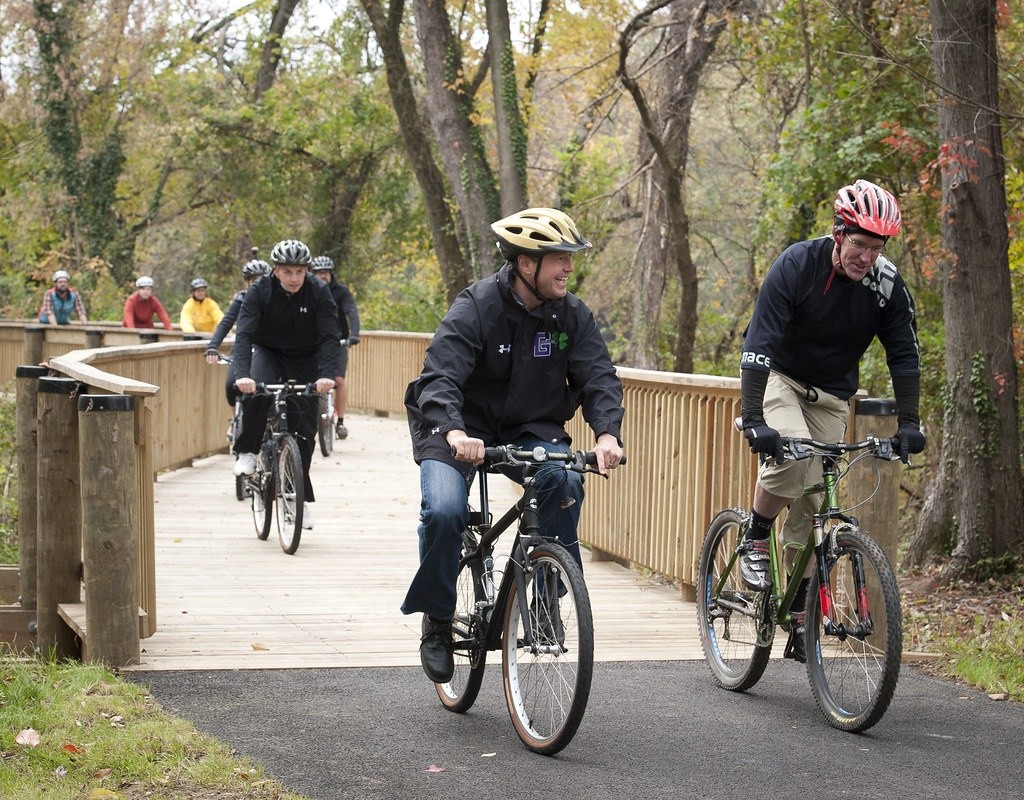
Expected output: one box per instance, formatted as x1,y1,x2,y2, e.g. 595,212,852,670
735,180,927,665
231,238,339,531
202,259,273,442
309,255,362,439
401,207,626,686
121,276,174,331
179,278,225,341
38,270,88,325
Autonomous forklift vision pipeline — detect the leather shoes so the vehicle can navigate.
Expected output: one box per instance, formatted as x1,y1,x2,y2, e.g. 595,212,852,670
530,596,566,644
420,613,454,684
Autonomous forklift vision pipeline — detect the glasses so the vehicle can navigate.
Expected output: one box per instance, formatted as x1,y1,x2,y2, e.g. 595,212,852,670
845,232,887,256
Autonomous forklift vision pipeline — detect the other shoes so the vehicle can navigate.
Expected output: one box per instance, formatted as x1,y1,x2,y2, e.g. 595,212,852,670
335,424,348,439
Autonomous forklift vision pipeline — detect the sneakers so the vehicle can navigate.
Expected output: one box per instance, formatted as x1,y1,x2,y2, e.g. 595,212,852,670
287,500,315,530
231,452,258,477
792,619,808,664
737,518,773,592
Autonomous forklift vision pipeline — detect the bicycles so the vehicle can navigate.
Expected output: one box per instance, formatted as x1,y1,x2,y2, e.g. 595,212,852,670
215,338,350,556
695,417,912,734
421,443,627,757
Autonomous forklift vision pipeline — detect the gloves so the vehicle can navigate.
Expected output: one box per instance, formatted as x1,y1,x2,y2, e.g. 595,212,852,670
739,368,785,465
890,369,926,464
346,336,360,348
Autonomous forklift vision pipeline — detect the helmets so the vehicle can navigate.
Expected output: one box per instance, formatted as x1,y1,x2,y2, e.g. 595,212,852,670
311,256,335,270
270,238,313,266
135,276,154,288
834,178,902,236
489,207,593,262
52,269,71,282
190,278,208,290
241,259,271,280
